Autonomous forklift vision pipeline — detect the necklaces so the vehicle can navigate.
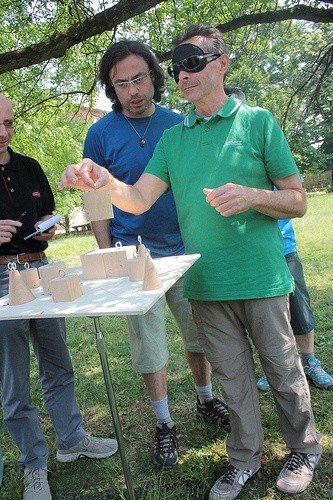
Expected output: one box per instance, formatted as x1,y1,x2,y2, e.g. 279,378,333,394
124,110,155,146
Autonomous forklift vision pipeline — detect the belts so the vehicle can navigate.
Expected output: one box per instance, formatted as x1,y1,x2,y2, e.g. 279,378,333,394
0,252,45,265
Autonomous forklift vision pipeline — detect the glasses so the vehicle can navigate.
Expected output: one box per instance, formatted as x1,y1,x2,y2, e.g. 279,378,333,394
0,120,14,128
110,70,151,89
167,52,223,78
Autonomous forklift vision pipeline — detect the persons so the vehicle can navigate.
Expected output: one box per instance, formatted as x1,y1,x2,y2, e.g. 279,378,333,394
82,40,233,468
0,93,118,500
221,86,333,391
58,24,324,500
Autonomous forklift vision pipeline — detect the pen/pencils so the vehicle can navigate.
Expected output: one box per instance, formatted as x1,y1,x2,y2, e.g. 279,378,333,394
17,211,27,222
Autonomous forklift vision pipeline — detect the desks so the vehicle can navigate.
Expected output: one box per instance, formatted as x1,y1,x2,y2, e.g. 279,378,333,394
0,253,202,500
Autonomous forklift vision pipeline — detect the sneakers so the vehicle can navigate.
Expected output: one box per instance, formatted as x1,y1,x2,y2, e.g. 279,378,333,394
209,463,261,500
153,419,179,469
196,393,231,430
56,434,118,462
304,357,333,390
23,464,52,500
276,452,322,494
256,375,270,391
